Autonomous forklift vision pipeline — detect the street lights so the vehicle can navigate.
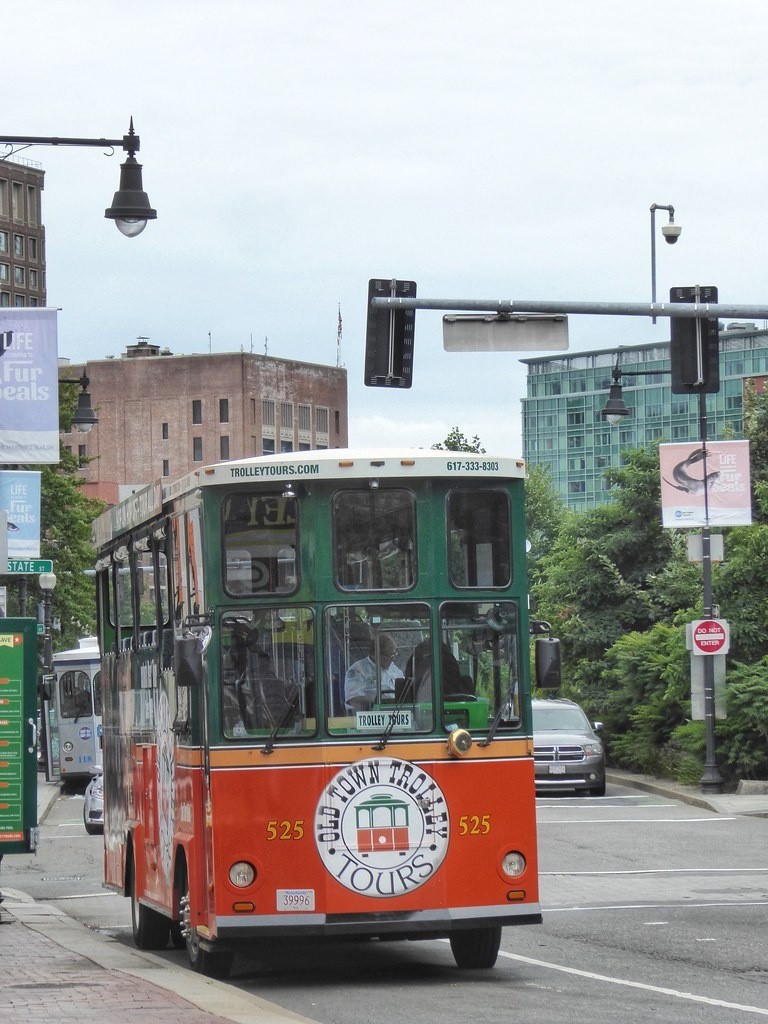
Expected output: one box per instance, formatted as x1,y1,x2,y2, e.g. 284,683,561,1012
601,368,727,795
37,572,58,773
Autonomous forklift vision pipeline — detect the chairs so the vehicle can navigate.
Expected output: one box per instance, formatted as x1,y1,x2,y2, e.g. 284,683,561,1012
224,642,293,730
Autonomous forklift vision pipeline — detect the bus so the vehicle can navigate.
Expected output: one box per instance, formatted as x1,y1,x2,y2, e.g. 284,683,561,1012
89,447,565,981
39,636,104,780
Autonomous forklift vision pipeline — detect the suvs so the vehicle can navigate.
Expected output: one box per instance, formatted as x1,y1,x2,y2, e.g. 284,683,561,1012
502,697,607,798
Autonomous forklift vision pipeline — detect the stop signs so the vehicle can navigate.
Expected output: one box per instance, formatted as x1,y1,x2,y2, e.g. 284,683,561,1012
693,620,726,653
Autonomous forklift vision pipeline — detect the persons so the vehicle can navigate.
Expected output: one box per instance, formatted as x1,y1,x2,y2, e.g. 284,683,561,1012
345,635,405,714
79,691,92,712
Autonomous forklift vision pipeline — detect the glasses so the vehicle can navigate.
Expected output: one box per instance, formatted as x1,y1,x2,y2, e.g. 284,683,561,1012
381,651,398,658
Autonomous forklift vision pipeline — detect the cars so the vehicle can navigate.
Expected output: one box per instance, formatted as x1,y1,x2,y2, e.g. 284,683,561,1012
83,764,104,836
